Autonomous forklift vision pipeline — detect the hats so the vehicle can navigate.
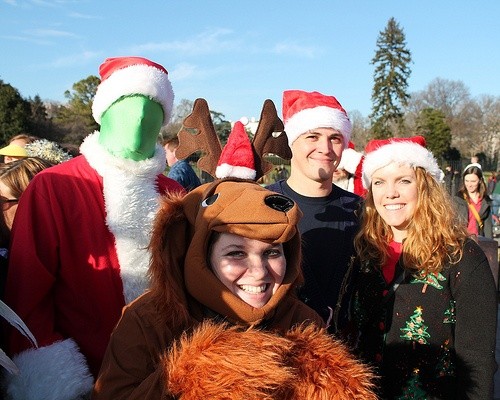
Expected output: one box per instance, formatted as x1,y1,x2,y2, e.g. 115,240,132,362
283,90,352,148
0,145,28,157
216,121,255,179
354,136,440,200
91,56,175,125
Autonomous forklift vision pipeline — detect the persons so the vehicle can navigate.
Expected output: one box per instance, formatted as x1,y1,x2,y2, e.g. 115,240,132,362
0,55,500,400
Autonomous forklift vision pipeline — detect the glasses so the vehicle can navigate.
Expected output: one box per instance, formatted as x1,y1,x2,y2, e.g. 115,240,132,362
0,195,18,210
